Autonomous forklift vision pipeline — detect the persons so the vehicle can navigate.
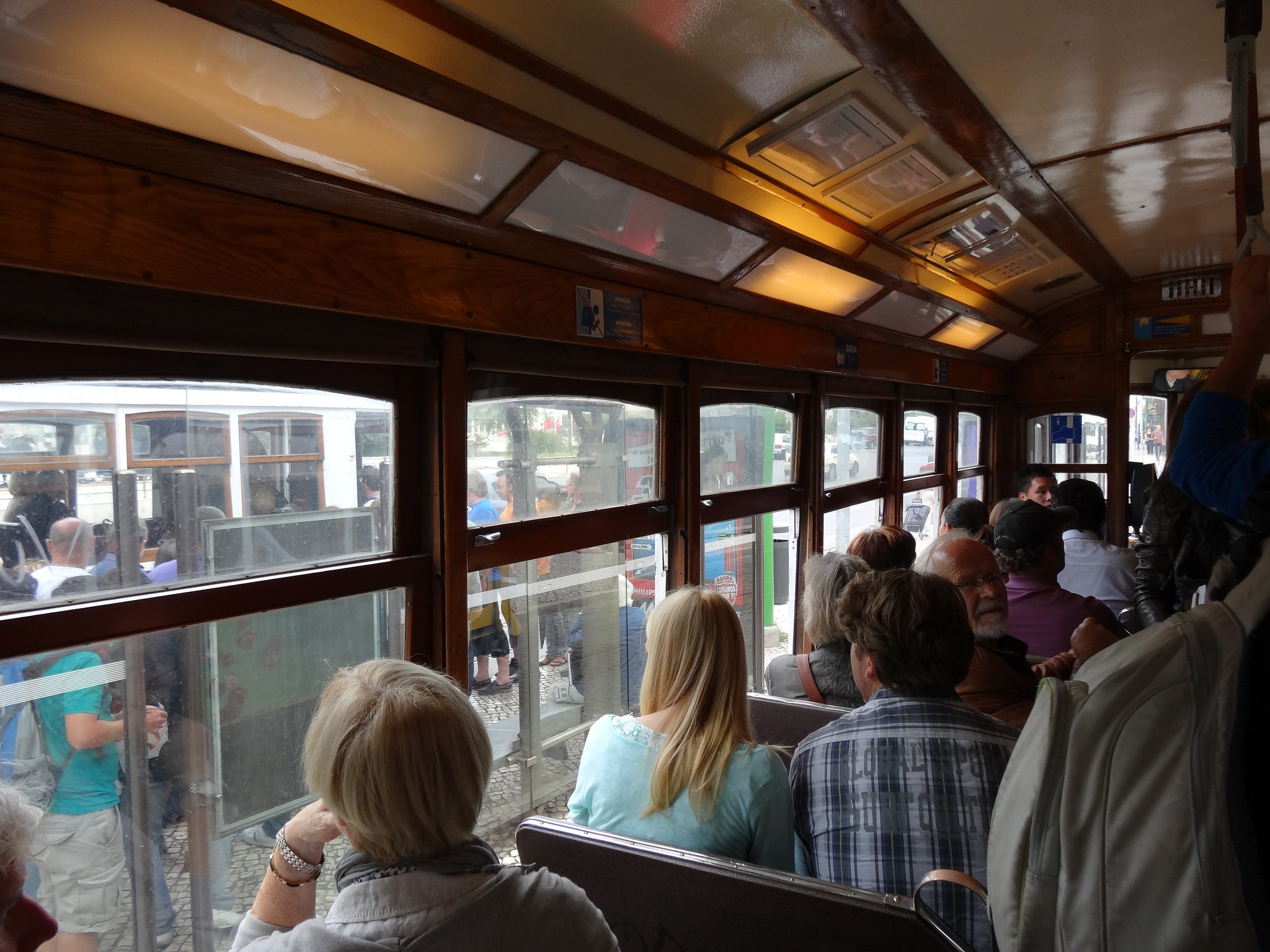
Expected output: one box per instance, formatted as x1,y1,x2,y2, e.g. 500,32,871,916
984,552,1270,952
566,587,794,876
788,568,1023,952
467,470,585,695
995,499,1130,657
916,531,1109,730
224,657,617,951
762,553,873,710
362,466,381,508
700,444,731,492
848,524,916,572
549,573,648,712
0,470,317,952
1050,478,1138,620
1128,252,1270,626
938,468,1057,549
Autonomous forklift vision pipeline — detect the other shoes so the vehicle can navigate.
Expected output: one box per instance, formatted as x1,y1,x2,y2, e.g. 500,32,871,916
495,658,518,678
478,680,513,695
509,672,518,683
473,676,492,689
542,744,568,760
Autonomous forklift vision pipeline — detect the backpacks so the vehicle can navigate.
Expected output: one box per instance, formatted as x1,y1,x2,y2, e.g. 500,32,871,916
989,536,1269,951
0,647,107,814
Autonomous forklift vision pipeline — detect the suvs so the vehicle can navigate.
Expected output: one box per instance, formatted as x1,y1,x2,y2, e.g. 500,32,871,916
849,427,877,449
774,433,859,480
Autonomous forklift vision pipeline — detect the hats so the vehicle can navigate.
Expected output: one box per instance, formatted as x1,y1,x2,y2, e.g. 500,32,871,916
993,499,1080,556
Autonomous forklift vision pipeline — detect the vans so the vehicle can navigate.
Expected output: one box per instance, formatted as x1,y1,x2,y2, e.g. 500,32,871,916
904,416,935,446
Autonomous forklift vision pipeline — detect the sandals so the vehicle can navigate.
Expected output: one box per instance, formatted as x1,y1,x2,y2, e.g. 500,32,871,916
549,658,567,666
539,657,552,666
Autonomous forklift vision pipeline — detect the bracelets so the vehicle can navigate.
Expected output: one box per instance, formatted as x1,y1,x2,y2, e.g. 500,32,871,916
270,851,321,887
276,822,325,873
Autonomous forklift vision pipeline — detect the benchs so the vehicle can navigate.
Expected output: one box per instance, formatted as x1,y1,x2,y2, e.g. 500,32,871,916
515,816,936,952
746,692,855,770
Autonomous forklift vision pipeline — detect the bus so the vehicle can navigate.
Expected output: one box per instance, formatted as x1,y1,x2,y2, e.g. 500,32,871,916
0,385,393,779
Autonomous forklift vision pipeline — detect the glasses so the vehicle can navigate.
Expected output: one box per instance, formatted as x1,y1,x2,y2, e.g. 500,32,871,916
954,572,1010,595
